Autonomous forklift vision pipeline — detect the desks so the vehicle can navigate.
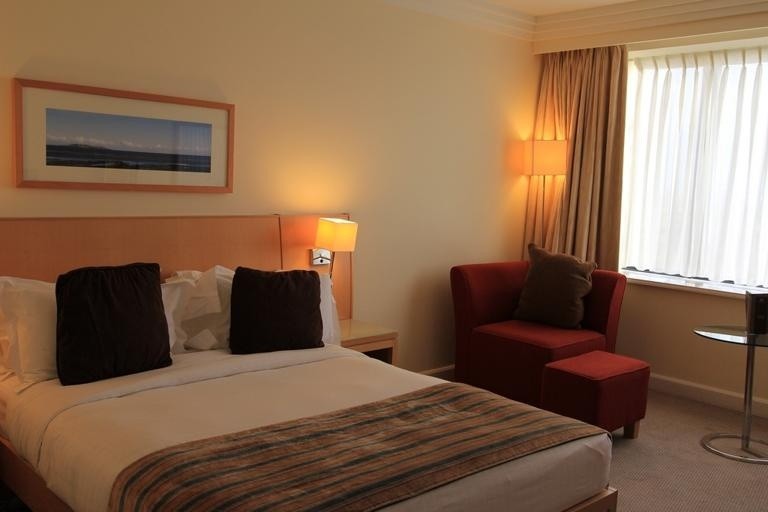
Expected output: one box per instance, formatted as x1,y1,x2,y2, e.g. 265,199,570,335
690,321,768,464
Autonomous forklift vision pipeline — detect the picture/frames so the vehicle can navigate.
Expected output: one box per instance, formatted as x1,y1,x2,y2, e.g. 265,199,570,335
12,77,236,192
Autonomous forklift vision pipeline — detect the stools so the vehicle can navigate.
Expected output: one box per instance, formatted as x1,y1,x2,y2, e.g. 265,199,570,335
540,349,650,442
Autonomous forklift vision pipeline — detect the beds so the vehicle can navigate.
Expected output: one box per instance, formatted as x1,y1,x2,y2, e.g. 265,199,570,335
0,216,619,511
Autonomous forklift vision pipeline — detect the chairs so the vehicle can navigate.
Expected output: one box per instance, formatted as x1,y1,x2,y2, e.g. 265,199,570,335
450,261,628,406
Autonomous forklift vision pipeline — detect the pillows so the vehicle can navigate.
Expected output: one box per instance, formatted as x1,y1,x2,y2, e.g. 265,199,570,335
513,243,598,330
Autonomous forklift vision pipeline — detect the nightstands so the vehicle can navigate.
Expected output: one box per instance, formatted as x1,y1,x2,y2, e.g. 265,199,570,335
338,318,399,374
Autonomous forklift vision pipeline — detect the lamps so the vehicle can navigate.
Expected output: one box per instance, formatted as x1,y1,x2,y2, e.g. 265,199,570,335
317,214,359,296
525,139,567,249
